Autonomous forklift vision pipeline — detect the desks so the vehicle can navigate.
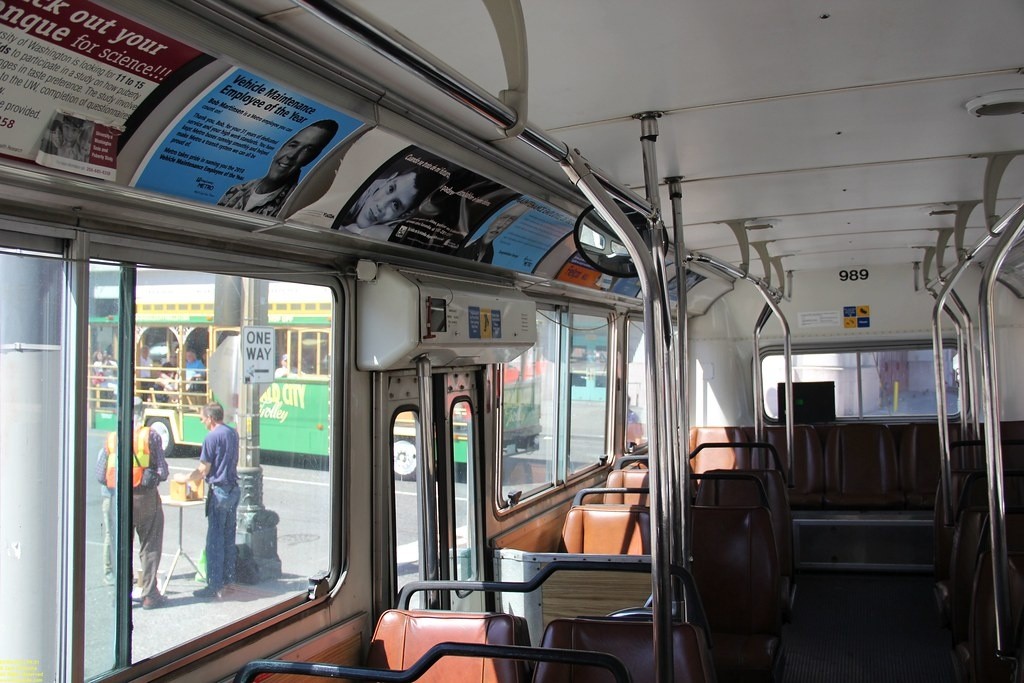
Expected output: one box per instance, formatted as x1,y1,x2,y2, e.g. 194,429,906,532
160,495,208,596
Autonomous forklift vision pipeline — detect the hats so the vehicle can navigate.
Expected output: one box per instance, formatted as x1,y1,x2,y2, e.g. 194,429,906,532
133,395,142,418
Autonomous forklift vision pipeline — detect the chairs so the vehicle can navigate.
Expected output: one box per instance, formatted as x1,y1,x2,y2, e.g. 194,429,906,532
694,468,791,577
363,609,529,683
558,487,649,556
932,439,1024,683
531,617,716,683
690,421,1024,511
689,474,786,683
603,455,650,509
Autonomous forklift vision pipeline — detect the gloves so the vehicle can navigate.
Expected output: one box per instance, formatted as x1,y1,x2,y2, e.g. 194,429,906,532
174,472,187,484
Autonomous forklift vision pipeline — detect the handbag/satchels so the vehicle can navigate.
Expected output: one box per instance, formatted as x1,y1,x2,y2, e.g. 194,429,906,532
234,543,259,585
142,468,157,489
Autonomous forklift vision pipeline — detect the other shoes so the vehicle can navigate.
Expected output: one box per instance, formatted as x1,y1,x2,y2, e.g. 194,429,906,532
222,577,231,584
104,574,138,586
143,593,167,609
193,584,223,598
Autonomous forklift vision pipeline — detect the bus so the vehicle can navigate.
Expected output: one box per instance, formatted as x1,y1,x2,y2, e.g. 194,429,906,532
85,283,543,481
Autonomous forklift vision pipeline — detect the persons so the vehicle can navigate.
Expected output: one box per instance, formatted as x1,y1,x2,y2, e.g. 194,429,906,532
342,165,429,239
176,400,242,599
97,396,171,609
218,119,339,217
40,113,94,163
275,353,305,377
93,345,208,398
463,203,528,265
